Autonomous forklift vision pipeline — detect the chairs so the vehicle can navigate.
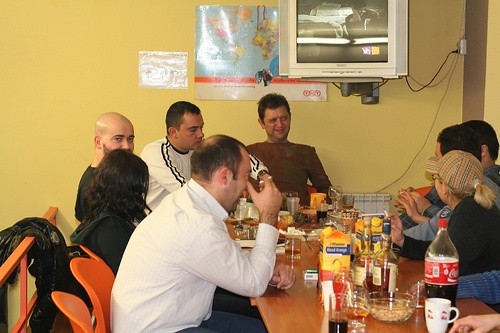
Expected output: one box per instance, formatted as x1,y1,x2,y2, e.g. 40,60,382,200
79,245,104,262
51,291,93,333
69,257,114,333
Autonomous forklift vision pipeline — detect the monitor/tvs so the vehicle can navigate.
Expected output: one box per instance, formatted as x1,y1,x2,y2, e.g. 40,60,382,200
278,0,408,82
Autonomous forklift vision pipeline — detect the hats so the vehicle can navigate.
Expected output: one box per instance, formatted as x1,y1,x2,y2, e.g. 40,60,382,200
426,149,484,193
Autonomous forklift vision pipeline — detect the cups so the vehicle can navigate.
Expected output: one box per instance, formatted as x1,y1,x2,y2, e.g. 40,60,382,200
284,235,302,261
233,197,260,240
348,288,370,328
341,192,355,212
287,197,300,216
316,203,328,223
408,281,425,308
424,298,460,333
277,211,290,240
332,268,355,322
310,193,326,210
329,293,350,333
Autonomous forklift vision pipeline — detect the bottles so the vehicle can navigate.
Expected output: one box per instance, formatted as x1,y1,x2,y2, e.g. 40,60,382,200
370,220,398,307
423,218,459,328
354,234,370,308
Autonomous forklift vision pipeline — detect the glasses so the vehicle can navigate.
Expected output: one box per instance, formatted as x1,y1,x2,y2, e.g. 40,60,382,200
432,174,439,181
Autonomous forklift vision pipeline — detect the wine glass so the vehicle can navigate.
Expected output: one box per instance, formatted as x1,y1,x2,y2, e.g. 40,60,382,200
328,185,343,216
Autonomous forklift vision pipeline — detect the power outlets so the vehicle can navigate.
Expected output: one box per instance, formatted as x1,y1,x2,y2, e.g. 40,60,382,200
458,38,467,56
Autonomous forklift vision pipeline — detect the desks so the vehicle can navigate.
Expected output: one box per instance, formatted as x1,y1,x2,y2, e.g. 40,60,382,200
225,219,500,333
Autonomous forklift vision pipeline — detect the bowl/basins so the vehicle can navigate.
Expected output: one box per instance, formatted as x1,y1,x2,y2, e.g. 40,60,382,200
366,291,417,323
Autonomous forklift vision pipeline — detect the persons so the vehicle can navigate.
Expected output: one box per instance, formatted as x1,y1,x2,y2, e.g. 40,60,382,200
384,119,500,333
240,93,332,222
79,112,135,183
132,101,205,226
70,149,151,278
110,134,283,333
